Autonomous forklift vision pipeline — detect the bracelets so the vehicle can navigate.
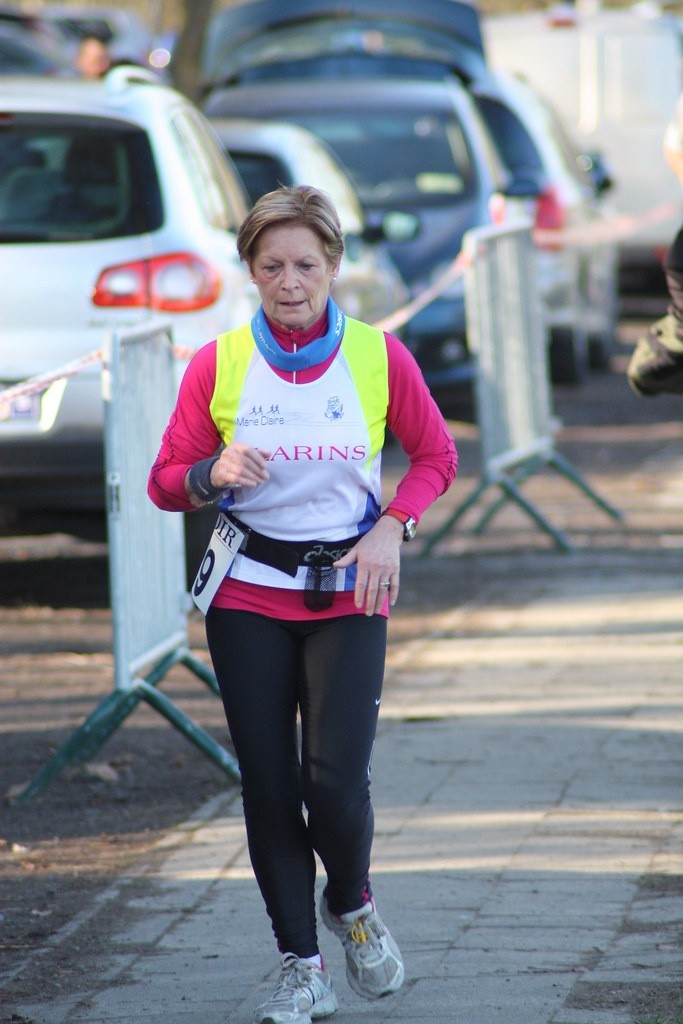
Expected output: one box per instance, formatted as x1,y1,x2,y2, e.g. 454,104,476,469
189,457,229,501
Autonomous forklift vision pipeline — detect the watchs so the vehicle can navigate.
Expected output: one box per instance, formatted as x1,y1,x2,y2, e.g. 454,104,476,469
378,508,417,542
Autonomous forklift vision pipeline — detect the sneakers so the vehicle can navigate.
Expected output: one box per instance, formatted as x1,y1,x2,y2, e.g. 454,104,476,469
319,888,405,1000
252,951,339,1024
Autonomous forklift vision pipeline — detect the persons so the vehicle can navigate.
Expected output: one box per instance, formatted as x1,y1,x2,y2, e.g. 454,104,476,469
627,92,683,399
72,38,110,81
146,186,459,1024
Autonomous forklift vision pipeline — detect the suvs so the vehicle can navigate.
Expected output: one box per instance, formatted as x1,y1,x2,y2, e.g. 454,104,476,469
1,0,625,412
0,64,260,563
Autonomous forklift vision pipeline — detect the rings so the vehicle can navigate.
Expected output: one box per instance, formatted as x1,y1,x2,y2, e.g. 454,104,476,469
379,582,390,589
